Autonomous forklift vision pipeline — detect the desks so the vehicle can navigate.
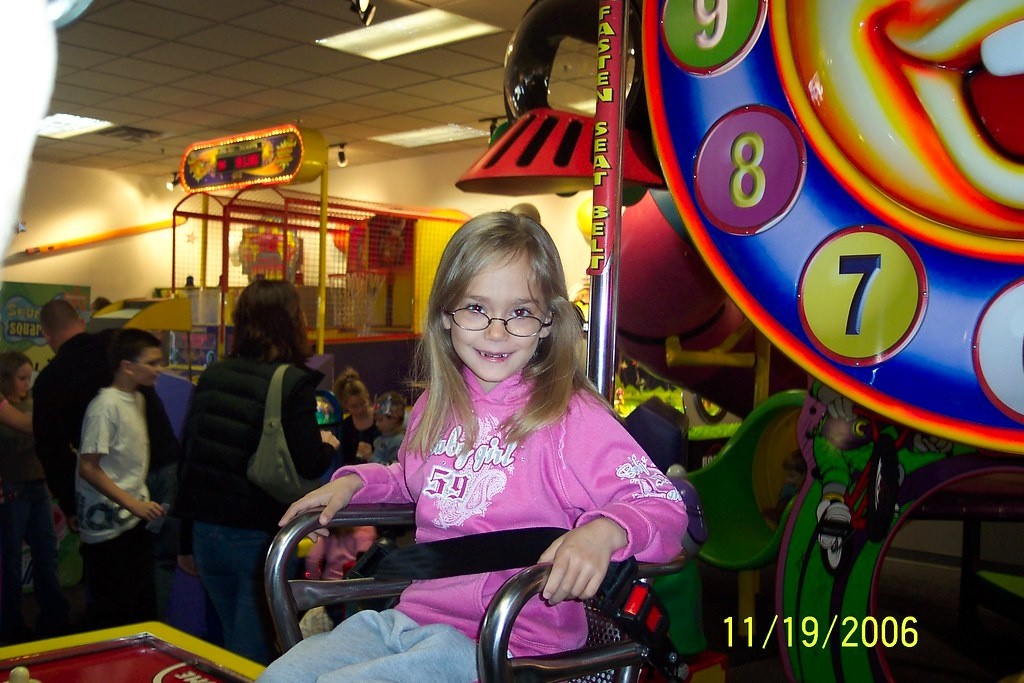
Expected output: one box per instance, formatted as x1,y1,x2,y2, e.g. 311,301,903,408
0,622,266,683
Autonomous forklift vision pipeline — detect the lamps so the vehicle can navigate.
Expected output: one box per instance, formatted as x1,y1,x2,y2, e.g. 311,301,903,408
351,0,378,27
166,171,182,191
337,143,348,168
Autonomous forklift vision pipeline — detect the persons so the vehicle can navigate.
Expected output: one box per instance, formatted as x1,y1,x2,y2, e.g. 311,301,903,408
177,278,341,667
0,392,33,435
0,350,71,643
334,368,405,468
257,211,690,683
75,327,167,631
31,299,179,565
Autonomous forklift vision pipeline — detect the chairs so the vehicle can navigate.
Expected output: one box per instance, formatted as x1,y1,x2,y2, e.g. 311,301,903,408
263,402,706,683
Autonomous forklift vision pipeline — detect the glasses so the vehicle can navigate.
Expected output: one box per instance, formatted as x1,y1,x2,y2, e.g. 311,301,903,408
444,308,555,337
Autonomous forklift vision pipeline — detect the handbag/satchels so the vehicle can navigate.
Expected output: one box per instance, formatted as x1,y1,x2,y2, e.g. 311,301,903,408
246,364,319,506
